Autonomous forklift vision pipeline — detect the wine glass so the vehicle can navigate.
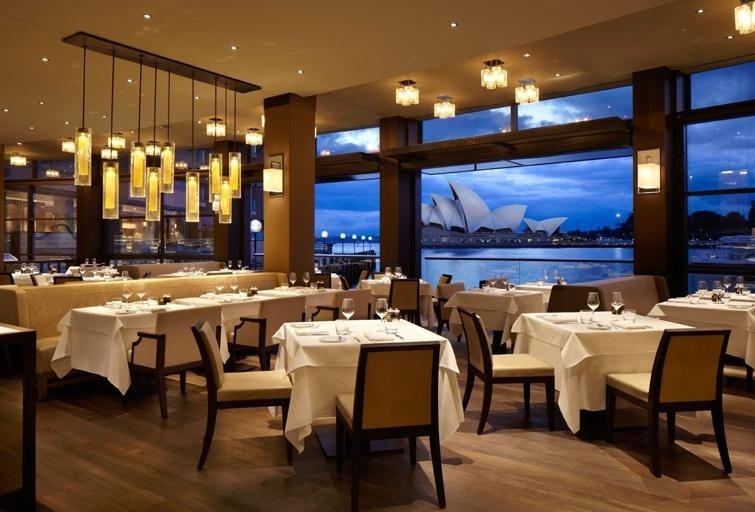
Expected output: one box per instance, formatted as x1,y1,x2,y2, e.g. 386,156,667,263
215,277,224,298
302,272,310,289
342,298,355,331
122,283,133,308
384,266,391,280
289,272,297,291
588,292,600,321
227,261,232,271
229,278,239,297
375,298,388,332
394,267,402,279
611,291,623,322
237,260,242,270
135,282,146,307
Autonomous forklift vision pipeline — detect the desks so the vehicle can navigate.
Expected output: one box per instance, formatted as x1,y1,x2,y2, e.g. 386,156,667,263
513,307,696,433
116,261,227,279
51,299,222,417
652,290,755,369
200,289,306,369
512,282,558,304
1,322,37,512
445,286,543,349
277,316,461,463
276,284,373,320
360,275,432,321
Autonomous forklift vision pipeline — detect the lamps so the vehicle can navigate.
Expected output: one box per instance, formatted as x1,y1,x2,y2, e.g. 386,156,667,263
175,161,188,171
218,105,233,223
11,152,27,166
243,124,265,148
184,78,201,223
46,169,62,178
395,59,540,120
101,148,118,160
161,83,174,195
146,141,163,157
106,137,127,150
206,116,228,141
130,76,148,197
208,83,223,206
61,137,77,153
144,92,162,223
261,167,283,196
72,52,94,188
734,0,755,34
100,84,121,220
228,97,242,199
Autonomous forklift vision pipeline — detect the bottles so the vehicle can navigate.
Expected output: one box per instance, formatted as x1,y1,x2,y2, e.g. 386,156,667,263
250,286,258,296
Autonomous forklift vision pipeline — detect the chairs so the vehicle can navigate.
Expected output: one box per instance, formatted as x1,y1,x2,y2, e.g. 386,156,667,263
328,275,351,292
453,305,556,436
335,338,448,509
430,273,453,321
191,320,295,469
53,276,83,284
9,272,35,286
601,330,734,478
30,273,49,286
547,284,605,316
478,278,508,289
386,279,421,326
437,281,465,337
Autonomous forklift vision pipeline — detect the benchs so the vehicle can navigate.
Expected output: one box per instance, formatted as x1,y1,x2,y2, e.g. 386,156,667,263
1,270,291,400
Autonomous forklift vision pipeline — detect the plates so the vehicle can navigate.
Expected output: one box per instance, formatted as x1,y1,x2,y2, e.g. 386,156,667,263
319,335,346,342
588,321,612,330
290,323,316,328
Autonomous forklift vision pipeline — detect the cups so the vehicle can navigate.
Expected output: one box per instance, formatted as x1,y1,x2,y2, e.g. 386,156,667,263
310,282,317,290
690,275,752,306
245,266,249,270
239,289,247,298
393,307,401,332
20,258,173,280
207,290,214,298
385,309,394,334
281,283,289,289
111,297,122,308
623,308,637,323
177,265,204,274
482,269,568,294
579,309,593,324
148,297,158,307
